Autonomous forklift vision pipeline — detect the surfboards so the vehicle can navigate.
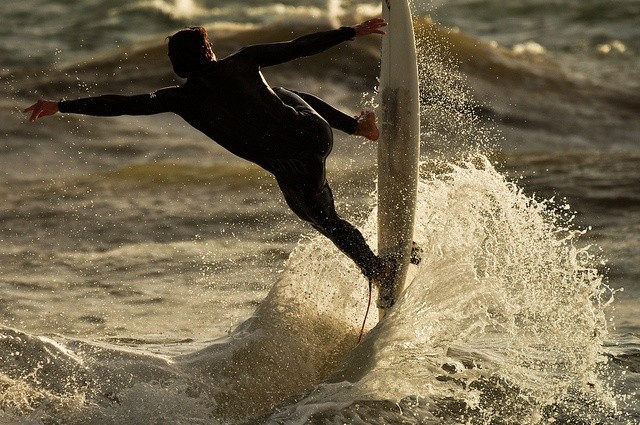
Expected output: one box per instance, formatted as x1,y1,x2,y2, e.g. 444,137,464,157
375,0,420,321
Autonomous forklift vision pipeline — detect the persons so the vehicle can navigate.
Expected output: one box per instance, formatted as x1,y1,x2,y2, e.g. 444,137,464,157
22,18,396,286
